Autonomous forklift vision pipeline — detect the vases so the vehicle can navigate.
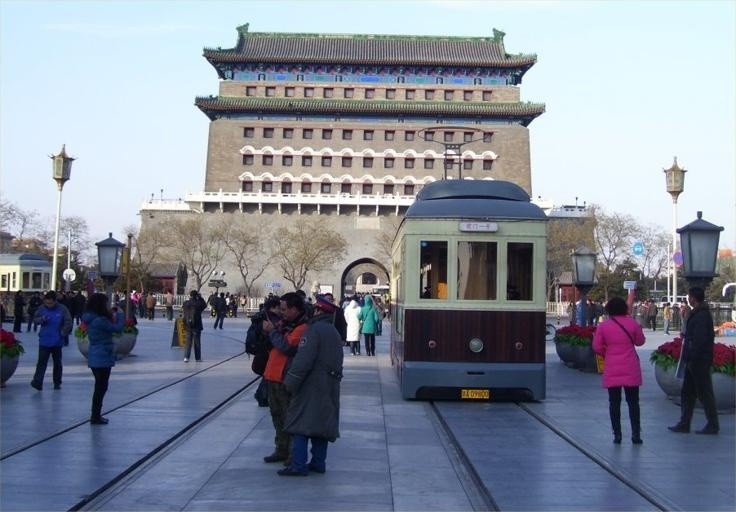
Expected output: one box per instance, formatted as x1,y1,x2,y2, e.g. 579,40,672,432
76,333,138,362
555,338,594,374
1,351,20,389
655,359,736,417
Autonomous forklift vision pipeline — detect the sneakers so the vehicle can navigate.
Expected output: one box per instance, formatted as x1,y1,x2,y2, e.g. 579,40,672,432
351,353,354,356
230,315,232,318
278,468,307,476
184,358,189,362
632,439,643,444
140,317,154,320
264,456,288,462
91,418,109,424
234,316,237,318
26,329,31,332
214,326,217,328
196,358,201,362
306,464,325,473
372,353,375,356
219,327,223,329
614,437,622,442
13,329,23,333
367,352,370,355
53,385,61,390
356,353,359,355
31,380,43,391
34,329,37,331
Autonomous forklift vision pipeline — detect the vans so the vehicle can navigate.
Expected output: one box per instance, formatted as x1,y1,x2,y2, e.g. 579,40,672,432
660,294,693,309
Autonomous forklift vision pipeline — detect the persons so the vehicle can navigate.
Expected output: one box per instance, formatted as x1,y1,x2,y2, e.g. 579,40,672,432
11,288,391,477
567,298,692,336
667,286,722,435
592,298,646,445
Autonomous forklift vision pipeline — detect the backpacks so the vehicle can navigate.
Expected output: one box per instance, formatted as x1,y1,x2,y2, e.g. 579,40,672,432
188,301,200,329
246,311,269,356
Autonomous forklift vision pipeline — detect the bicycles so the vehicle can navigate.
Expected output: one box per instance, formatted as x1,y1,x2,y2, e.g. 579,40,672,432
546,322,558,343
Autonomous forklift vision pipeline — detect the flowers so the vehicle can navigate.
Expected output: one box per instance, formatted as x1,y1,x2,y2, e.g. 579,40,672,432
75,315,139,336
554,323,597,346
0,329,26,359
650,336,736,376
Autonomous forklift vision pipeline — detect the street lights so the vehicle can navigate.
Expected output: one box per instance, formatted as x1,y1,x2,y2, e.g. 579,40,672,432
676,208,725,302
95,233,125,308
212,269,224,294
664,153,688,310
47,142,78,291
570,246,599,327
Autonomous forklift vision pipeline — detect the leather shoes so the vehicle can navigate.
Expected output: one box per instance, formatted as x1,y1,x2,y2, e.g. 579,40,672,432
696,428,719,433
668,425,690,433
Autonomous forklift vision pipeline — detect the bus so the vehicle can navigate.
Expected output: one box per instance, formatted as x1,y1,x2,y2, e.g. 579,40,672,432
722,282,736,324
0,252,53,324
371,286,389,296
387,179,548,404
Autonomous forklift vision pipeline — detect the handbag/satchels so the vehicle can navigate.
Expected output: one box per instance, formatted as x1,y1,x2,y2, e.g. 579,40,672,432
375,320,382,335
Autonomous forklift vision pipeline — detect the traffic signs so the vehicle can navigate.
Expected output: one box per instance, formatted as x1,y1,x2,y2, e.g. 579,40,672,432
263,282,280,288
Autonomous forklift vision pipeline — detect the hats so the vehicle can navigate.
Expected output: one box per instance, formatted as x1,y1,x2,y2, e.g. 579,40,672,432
313,295,341,313
